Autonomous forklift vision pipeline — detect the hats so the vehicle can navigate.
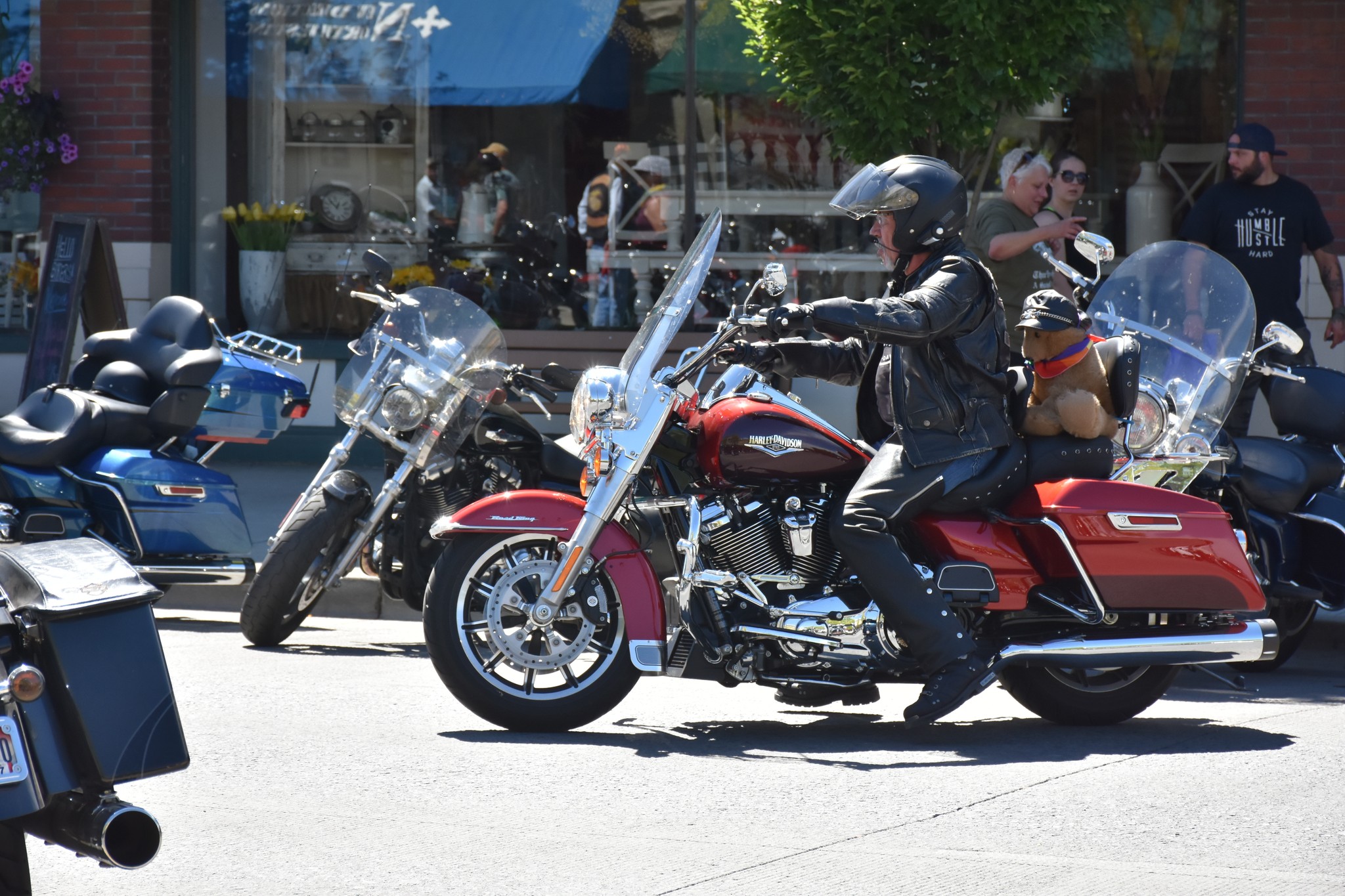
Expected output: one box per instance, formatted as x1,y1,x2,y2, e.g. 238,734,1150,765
632,155,669,177
1009,288,1092,335
1227,124,1289,157
480,142,510,165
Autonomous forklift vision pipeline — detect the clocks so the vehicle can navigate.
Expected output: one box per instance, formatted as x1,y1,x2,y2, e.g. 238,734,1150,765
310,183,364,233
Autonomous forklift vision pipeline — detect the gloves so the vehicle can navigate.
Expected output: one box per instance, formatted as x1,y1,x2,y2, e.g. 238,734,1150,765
713,338,748,366
766,303,812,336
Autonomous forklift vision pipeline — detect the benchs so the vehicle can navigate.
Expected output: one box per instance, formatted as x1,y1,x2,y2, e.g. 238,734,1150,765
600,248,899,324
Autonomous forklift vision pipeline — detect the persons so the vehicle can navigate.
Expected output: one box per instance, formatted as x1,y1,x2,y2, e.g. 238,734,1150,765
1179,122,1345,530
1032,145,1100,313
479,142,523,328
577,142,676,331
414,157,460,266
966,143,1087,358
716,155,1017,729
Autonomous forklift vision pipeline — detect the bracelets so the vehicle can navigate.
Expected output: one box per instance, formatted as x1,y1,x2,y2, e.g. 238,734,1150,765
1184,309,1202,317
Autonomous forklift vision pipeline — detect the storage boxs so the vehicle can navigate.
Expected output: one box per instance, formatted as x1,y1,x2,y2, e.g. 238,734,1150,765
1297,483,1345,608
195,343,312,446
1020,474,1270,614
76,442,257,560
0,532,193,791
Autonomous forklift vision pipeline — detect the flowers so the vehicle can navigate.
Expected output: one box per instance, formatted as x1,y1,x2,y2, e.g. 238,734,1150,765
0,44,80,193
219,199,307,251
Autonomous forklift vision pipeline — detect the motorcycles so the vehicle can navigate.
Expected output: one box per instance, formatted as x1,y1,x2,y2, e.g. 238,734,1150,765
0,540,194,894
1078,240,1345,671
236,249,707,646
1,297,313,587
420,210,1283,735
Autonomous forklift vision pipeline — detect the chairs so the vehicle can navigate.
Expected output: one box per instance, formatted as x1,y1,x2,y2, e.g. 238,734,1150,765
0,229,41,331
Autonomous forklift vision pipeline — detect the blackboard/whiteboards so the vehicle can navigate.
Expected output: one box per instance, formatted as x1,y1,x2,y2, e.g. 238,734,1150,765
16,212,130,407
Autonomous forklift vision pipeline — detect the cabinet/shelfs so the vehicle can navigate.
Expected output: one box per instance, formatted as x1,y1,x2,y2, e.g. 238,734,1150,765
244,5,435,277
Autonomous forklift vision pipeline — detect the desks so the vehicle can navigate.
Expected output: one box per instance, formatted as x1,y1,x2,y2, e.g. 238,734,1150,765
658,185,1001,271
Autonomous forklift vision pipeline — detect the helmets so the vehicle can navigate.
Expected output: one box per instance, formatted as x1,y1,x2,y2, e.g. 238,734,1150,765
478,153,500,171
829,154,967,260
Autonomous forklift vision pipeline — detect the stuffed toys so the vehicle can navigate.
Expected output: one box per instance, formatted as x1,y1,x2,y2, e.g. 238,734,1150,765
1014,287,1119,439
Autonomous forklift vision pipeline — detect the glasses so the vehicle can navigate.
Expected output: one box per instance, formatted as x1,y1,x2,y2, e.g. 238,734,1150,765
1012,150,1038,177
1058,168,1088,186
873,211,895,228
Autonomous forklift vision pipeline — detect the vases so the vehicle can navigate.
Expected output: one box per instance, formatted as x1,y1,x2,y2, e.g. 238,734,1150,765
239,247,288,338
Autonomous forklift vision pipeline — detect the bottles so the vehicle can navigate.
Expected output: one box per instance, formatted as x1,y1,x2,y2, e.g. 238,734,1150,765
285,105,405,143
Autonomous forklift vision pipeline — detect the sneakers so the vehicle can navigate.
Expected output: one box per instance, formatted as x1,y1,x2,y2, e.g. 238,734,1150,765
904,648,997,727
774,681,881,707
